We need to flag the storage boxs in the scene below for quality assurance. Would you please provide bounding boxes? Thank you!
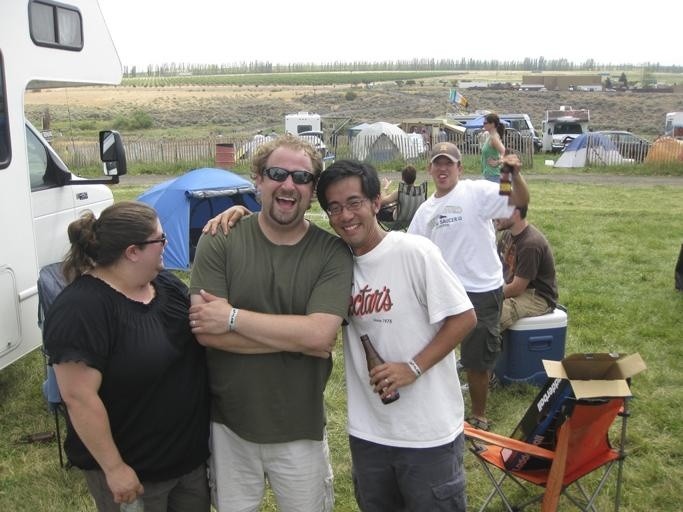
[494,308,568,386]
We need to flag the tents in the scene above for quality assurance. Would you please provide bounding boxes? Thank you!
[135,167,262,271]
[351,122,418,162]
[553,133,623,168]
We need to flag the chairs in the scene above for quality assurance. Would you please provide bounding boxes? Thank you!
[464,378,631,512]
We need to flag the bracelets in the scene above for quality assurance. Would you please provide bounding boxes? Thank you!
[228,308,239,332]
[407,360,422,377]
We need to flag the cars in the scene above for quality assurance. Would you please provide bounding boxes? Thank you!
[299,133,329,157]
[597,129,652,162]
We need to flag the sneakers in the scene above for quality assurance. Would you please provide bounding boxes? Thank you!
[465,418,490,440]
[461,370,500,393]
[456,358,467,371]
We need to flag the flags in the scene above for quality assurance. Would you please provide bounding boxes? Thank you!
[449,88,468,108]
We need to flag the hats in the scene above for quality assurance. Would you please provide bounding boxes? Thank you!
[430,141,462,163]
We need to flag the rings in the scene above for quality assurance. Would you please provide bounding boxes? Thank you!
[192,321,195,326]
[385,379,390,384]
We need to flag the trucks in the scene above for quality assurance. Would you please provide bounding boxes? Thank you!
[665,111,683,141]
[451,108,592,157]
[0,0,133,378]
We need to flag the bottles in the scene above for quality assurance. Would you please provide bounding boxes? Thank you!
[358,334,402,407]
[498,149,512,198]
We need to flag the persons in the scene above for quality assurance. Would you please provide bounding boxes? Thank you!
[40,201,211,512]
[188,133,354,512]
[413,127,447,145]
[203,159,478,512]
[457,204,558,391]
[376,166,416,221]
[480,113,506,183]
[408,141,529,431]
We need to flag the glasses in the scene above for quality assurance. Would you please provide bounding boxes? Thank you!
[125,232,167,249]
[262,166,321,185]
[326,197,370,216]
[484,121,489,125]
